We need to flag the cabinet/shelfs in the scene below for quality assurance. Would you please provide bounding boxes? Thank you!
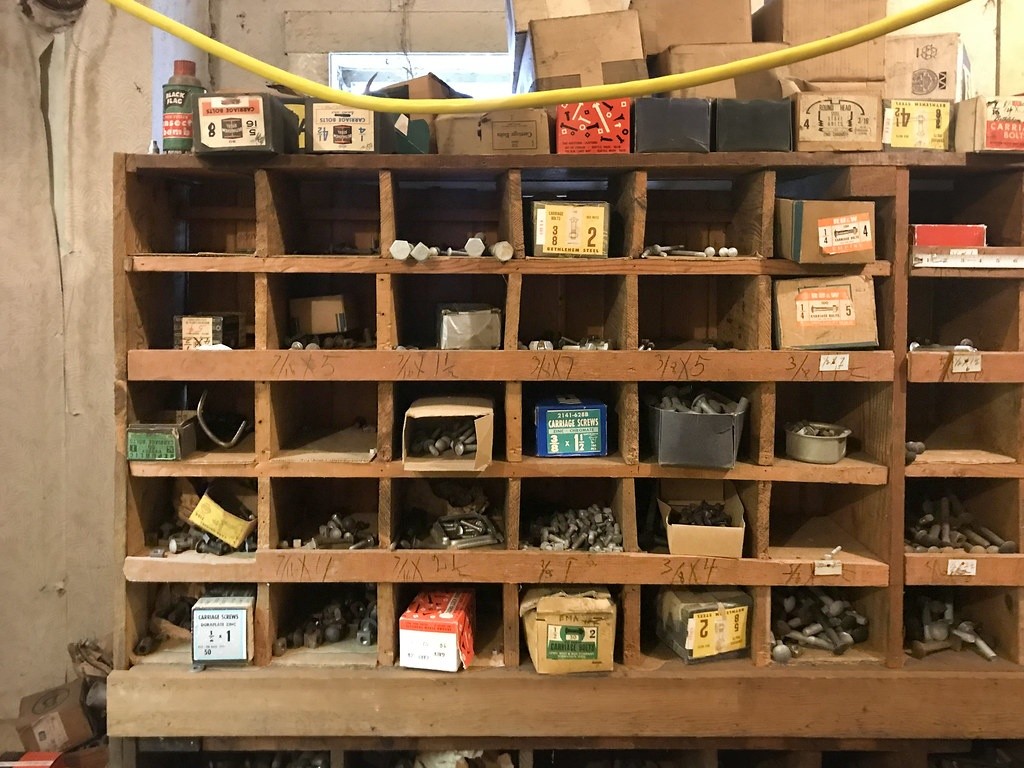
[107,145,1024,768]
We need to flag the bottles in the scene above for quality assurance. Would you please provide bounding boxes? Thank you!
[162,60,203,151]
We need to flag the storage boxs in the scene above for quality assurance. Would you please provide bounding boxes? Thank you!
[192,591,253,665]
[126,408,204,461]
[531,200,611,259]
[656,479,746,557]
[289,293,357,336]
[777,195,880,268]
[173,311,245,350]
[535,393,608,457]
[649,394,749,468]
[766,275,884,353]
[910,223,989,250]
[160,477,259,549]
[192,0,1024,154]
[656,587,754,664]
[401,392,493,471]
[519,587,617,673]
[399,588,475,672]
[434,302,502,350]
